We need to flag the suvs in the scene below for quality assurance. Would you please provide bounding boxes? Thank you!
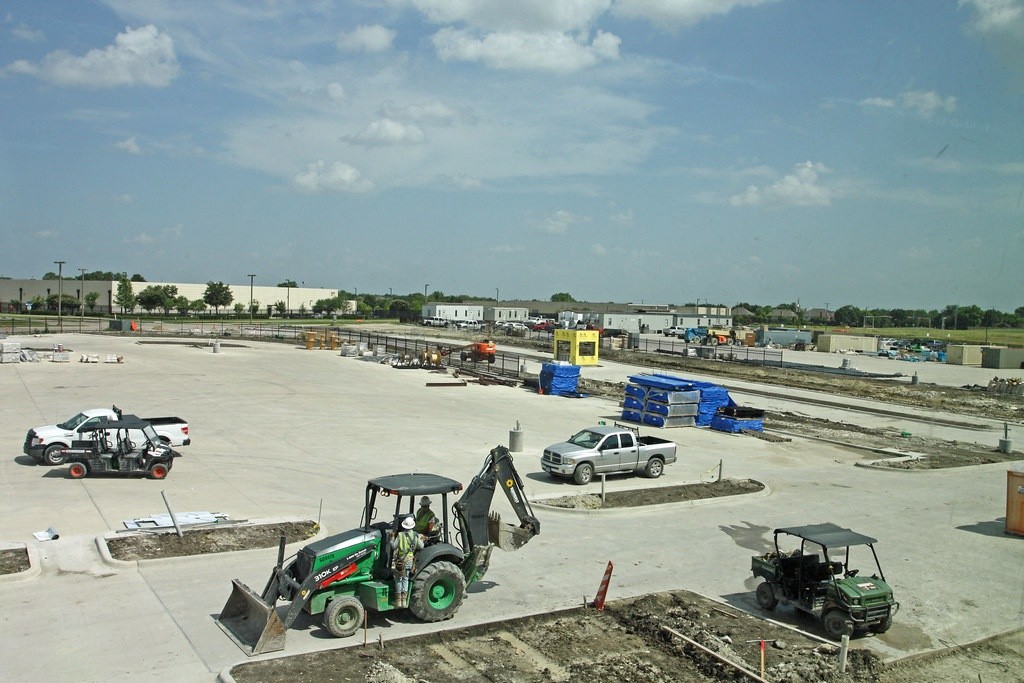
[603,328,632,337]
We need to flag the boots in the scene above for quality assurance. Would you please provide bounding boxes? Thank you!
[401,592,408,608]
[392,593,402,608]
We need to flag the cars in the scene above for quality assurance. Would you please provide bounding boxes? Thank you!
[490,320,529,332]
[874,337,967,351]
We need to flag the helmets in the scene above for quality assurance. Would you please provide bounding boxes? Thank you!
[401,516,416,529]
[419,496,432,506]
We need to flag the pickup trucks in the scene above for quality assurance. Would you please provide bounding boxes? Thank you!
[578,324,604,338]
[421,316,450,327]
[22,405,191,464]
[663,326,686,337]
[456,320,487,331]
[532,321,554,331]
[541,418,678,486]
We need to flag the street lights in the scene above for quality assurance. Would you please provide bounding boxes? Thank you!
[247,274,257,323]
[54,261,66,326]
[285,279,290,319]
[77,268,87,321]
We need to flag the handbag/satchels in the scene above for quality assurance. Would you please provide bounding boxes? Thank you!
[394,559,406,576]
[410,557,418,575]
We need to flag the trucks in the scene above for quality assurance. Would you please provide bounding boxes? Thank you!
[752,523,900,641]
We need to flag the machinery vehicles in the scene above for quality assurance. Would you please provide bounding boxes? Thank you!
[436,336,498,365]
[212,443,541,657]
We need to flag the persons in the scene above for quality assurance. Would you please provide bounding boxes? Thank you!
[413,496,435,533]
[389,517,424,607]
